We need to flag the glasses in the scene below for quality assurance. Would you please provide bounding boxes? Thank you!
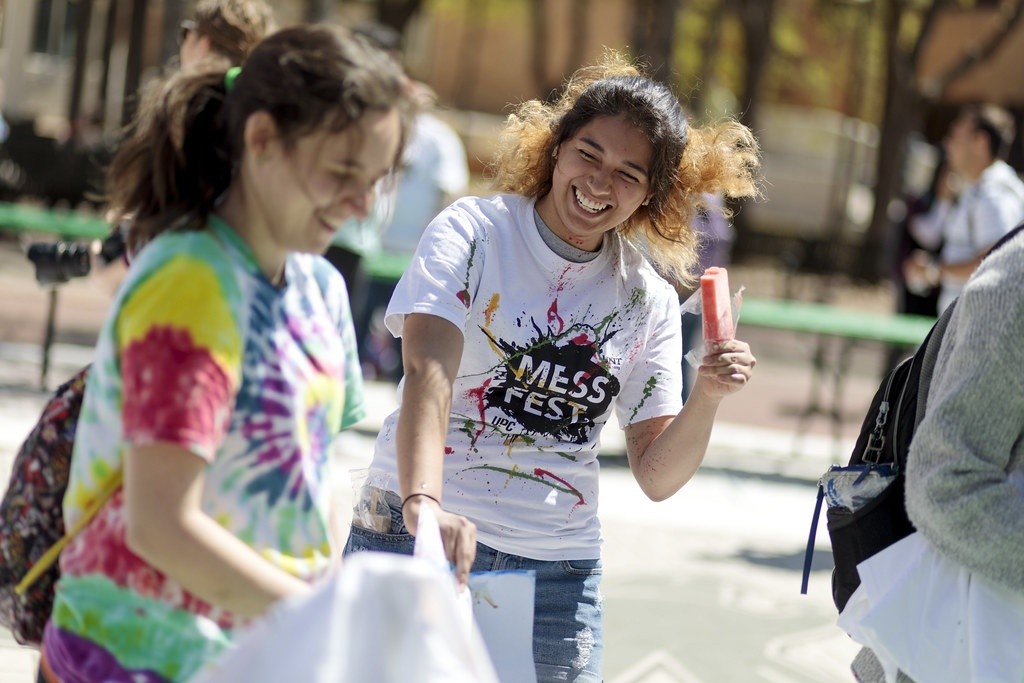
[175,20,197,47]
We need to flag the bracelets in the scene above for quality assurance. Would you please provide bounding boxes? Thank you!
[397,495,439,518]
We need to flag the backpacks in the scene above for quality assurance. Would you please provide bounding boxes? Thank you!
[0,365,122,643]
[820,220,1024,616]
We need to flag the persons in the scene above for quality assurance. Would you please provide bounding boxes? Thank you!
[332,81,479,387]
[910,105,1023,316]
[847,218,1023,683]
[175,1,269,75]
[657,175,724,408]
[36,20,416,683]
[345,62,757,683]
